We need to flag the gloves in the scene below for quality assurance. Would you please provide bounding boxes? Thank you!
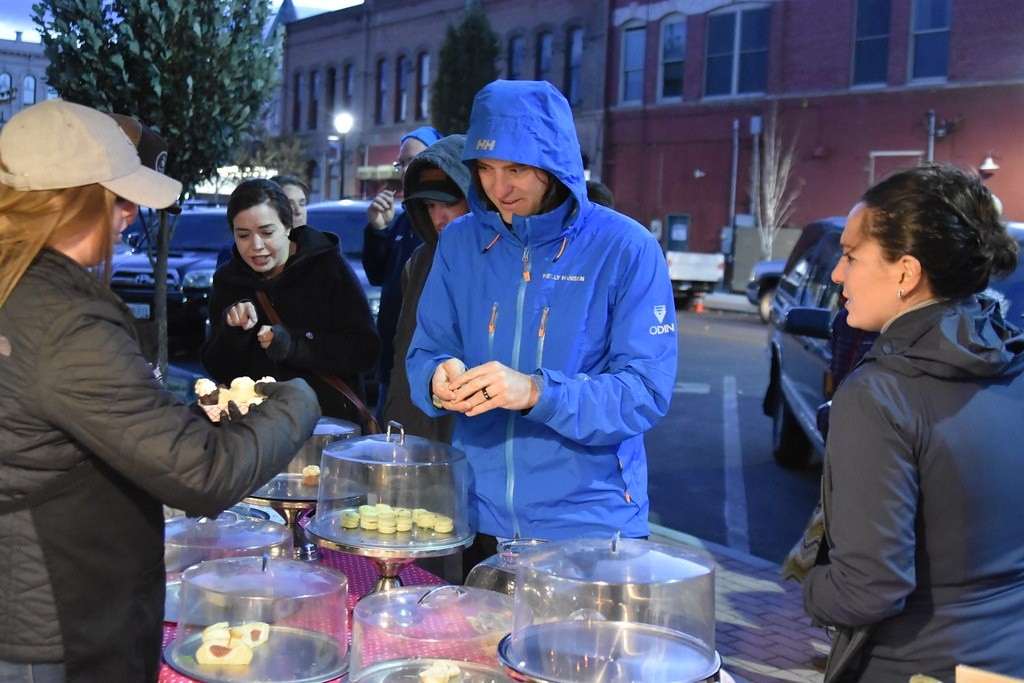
[219,400,257,425]
[255,378,323,415]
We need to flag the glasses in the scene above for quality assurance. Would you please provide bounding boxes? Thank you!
[392,160,411,173]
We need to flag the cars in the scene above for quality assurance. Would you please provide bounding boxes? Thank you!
[303,196,403,326]
[83,199,237,306]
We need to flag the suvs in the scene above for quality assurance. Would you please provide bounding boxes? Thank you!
[761,214,1024,466]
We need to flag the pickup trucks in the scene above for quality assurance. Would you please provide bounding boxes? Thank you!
[662,248,726,312]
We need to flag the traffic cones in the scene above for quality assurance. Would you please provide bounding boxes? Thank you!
[693,298,705,314]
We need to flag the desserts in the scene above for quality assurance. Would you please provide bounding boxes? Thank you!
[420,660,460,683]
[194,376,278,405]
[299,464,454,534]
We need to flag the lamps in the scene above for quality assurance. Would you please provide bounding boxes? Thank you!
[977,148,1000,170]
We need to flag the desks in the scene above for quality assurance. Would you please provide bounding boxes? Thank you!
[157,507,530,683]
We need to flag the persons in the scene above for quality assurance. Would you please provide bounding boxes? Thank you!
[103,114,182,224]
[362,126,446,422]
[201,179,381,437]
[404,79,678,586]
[382,134,472,446]
[801,163,1024,683]
[215,174,309,264]
[0,97,322,683]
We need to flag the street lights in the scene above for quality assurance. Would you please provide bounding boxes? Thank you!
[333,110,354,200]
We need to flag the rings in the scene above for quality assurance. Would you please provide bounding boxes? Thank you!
[482,388,491,400]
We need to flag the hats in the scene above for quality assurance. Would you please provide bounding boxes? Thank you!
[0,98,183,210]
[116,124,183,214]
[402,164,464,203]
[585,181,614,206]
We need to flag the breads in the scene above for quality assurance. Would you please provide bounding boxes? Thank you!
[194,622,270,665]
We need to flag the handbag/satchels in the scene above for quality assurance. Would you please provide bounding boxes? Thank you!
[363,415,383,435]
